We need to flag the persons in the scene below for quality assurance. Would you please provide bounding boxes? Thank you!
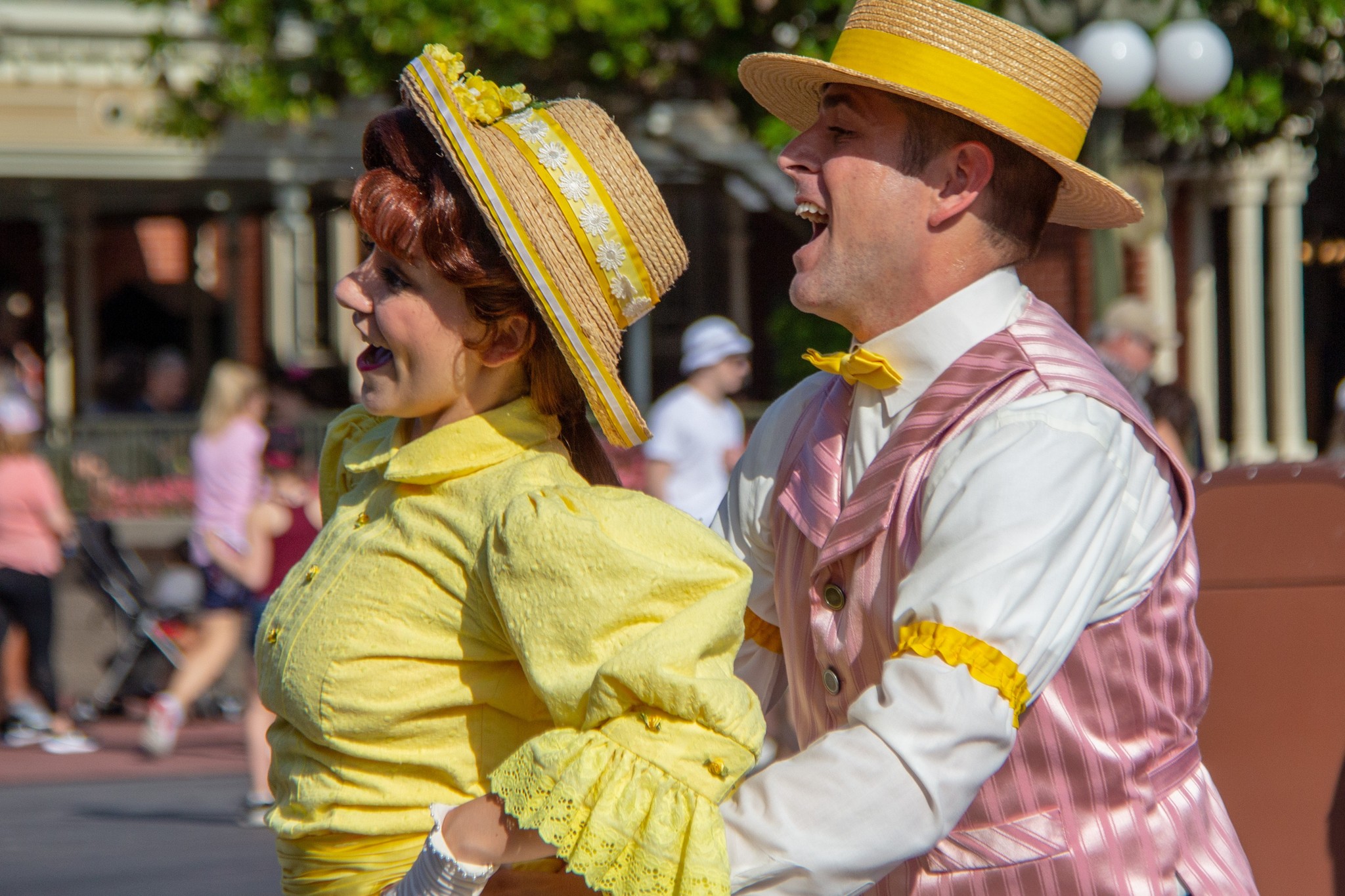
[641,316,755,530]
[144,360,270,756]
[253,44,768,896]
[1090,291,1209,478]
[197,433,323,826]
[481,1,1256,895]
[0,392,97,754]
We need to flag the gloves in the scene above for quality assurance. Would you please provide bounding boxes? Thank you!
[381,804,499,895]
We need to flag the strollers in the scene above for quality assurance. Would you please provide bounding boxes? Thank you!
[62,510,246,729]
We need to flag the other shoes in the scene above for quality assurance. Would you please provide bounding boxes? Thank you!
[140,692,185,763]
[43,727,100,754]
[7,720,58,748]
[241,792,279,830]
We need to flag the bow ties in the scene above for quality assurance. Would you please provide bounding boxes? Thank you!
[801,342,903,391]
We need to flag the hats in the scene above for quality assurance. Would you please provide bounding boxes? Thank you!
[1103,293,1164,352]
[400,42,691,451]
[736,0,1146,231]
[680,316,754,373]
